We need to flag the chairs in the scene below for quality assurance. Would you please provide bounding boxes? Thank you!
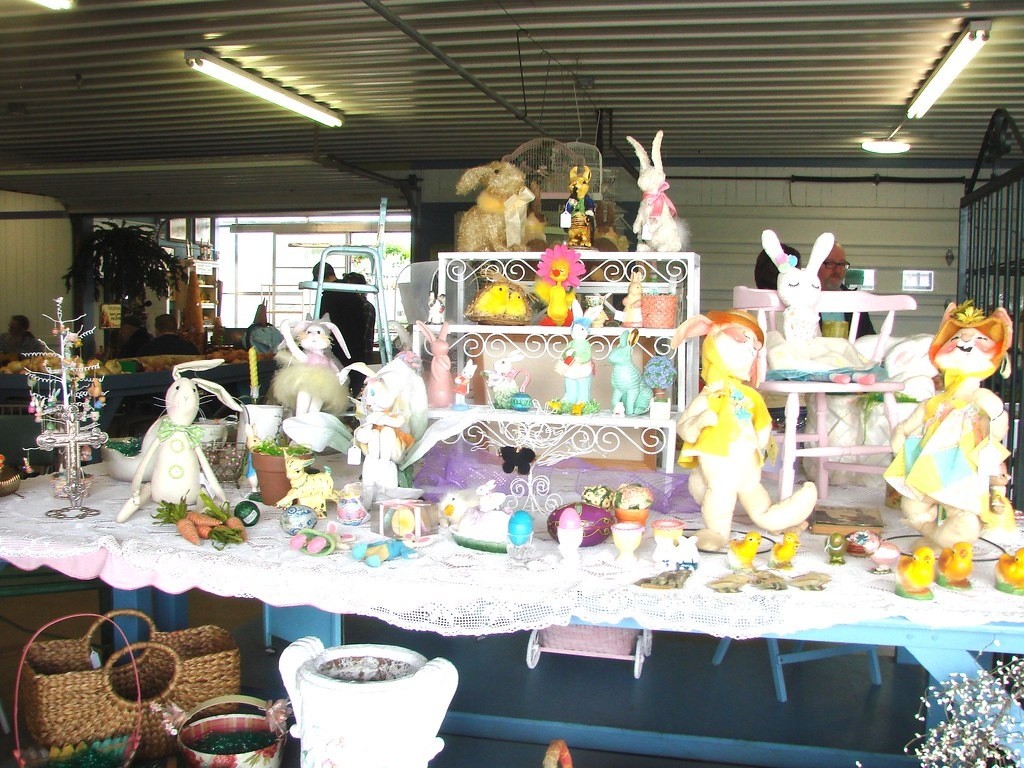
[732,285,916,504]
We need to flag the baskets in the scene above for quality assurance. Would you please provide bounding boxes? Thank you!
[641,296,677,329]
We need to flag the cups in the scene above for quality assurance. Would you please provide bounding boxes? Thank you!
[614,509,649,527]
[558,525,584,562]
[653,520,685,547]
[584,295,604,308]
[506,532,533,562]
[822,321,849,340]
[611,523,644,564]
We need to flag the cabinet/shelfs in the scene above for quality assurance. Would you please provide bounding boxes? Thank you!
[175,258,220,350]
[413,251,701,510]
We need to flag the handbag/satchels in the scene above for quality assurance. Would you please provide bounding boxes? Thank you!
[20,610,240,768]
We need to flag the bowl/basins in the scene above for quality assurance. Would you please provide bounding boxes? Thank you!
[101,447,157,481]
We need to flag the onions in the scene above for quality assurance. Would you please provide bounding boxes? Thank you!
[0,349,274,376]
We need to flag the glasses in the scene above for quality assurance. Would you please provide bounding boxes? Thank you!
[823,259,850,271]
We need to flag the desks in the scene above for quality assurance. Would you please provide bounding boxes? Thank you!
[0,460,1024,768]
[0,358,284,464]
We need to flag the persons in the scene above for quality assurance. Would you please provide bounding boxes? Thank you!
[0,314,199,367]
[312,262,376,434]
[754,240,877,433]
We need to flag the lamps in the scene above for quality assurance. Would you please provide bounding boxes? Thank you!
[186,52,348,135]
[906,20,995,121]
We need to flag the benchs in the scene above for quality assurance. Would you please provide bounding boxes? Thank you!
[126,355,205,369]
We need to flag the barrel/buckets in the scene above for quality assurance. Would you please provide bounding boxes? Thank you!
[177,695,289,768]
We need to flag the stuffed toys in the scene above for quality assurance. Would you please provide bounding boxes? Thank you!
[456,161,551,252]
[438,488,481,528]
[351,533,433,568]
[289,521,356,558]
[626,130,691,252]
[476,479,506,512]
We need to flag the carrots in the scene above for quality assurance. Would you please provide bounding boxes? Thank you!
[175,511,248,547]
